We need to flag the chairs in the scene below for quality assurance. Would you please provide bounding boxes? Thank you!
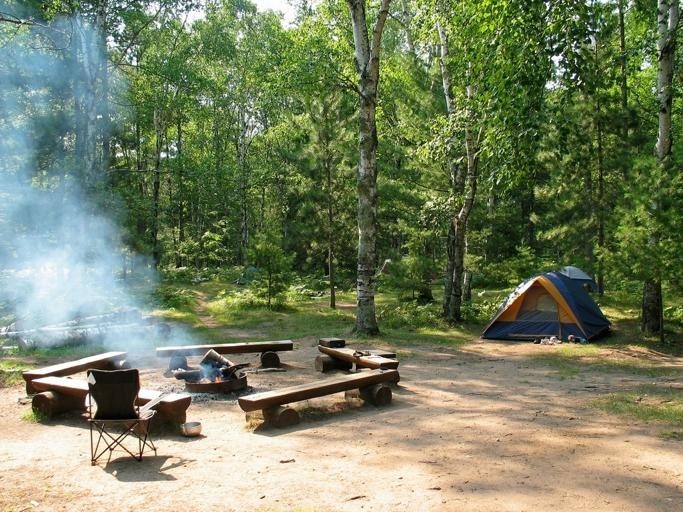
[82,368,161,466]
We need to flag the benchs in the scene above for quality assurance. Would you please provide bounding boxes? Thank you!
[29,376,191,426]
[19,350,130,396]
[239,368,402,427]
[321,337,400,370]
[154,340,293,375]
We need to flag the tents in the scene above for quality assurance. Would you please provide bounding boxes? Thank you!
[480,266,613,343]
